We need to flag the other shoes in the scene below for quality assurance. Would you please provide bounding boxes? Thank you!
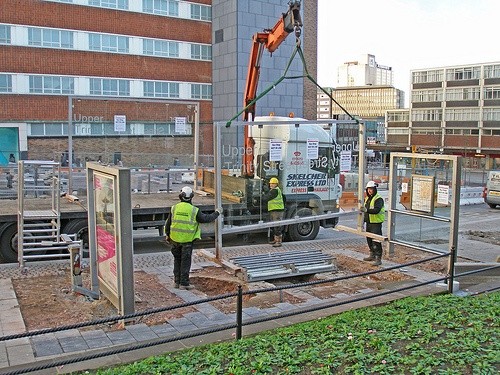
[269,235,282,247]
[363,251,376,261]
[173,282,195,290]
[371,256,382,266]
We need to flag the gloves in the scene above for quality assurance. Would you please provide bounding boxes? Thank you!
[360,206,368,213]
[215,206,223,215]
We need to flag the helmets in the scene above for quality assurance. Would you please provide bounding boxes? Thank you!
[268,177,279,184]
[367,181,377,188]
[181,186,192,198]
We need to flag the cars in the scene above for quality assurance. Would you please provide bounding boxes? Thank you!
[482,171,500,208]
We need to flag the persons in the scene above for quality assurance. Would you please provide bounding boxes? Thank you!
[82,156,88,167]
[258,177,285,247]
[360,181,385,266]
[61,153,66,167]
[174,156,181,166]
[164,186,223,290]
[75,158,80,168]
[97,156,102,162]
[116,158,123,167]
[6,172,13,188]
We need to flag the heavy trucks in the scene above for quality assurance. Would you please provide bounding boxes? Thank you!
[0,0,343,265]
